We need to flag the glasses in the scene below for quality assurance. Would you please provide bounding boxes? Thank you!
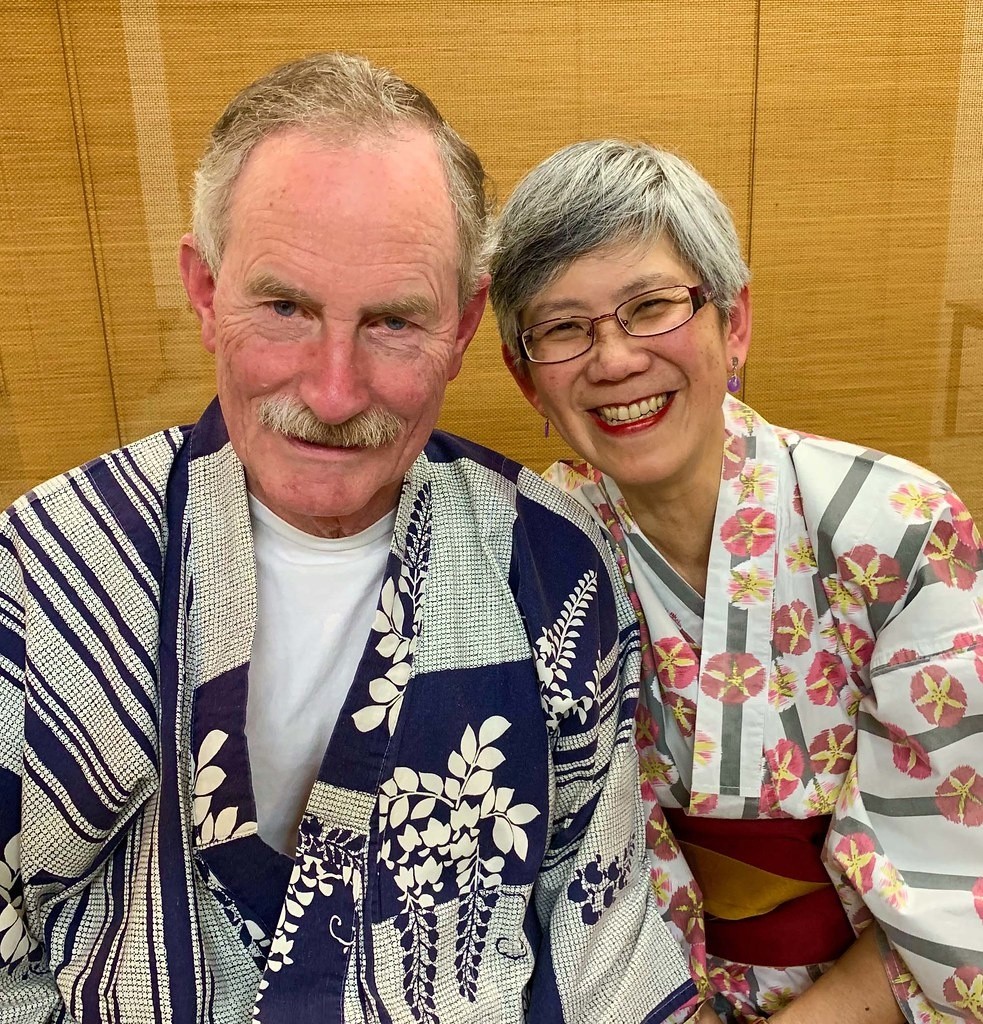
[518,282,713,364]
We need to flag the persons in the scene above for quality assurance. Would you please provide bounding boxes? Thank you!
[0,54,695,1024]
[494,141,983,1024]
